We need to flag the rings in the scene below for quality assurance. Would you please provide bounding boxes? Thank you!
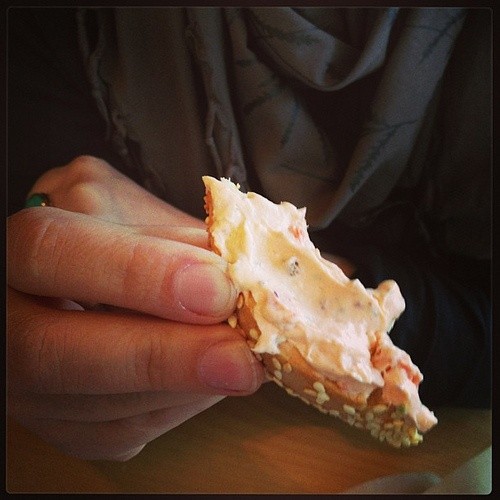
[22,190,53,212]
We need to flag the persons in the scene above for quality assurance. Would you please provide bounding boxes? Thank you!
[0,0,494,472]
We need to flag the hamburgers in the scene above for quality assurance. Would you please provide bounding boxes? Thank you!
[199,172,438,450]
